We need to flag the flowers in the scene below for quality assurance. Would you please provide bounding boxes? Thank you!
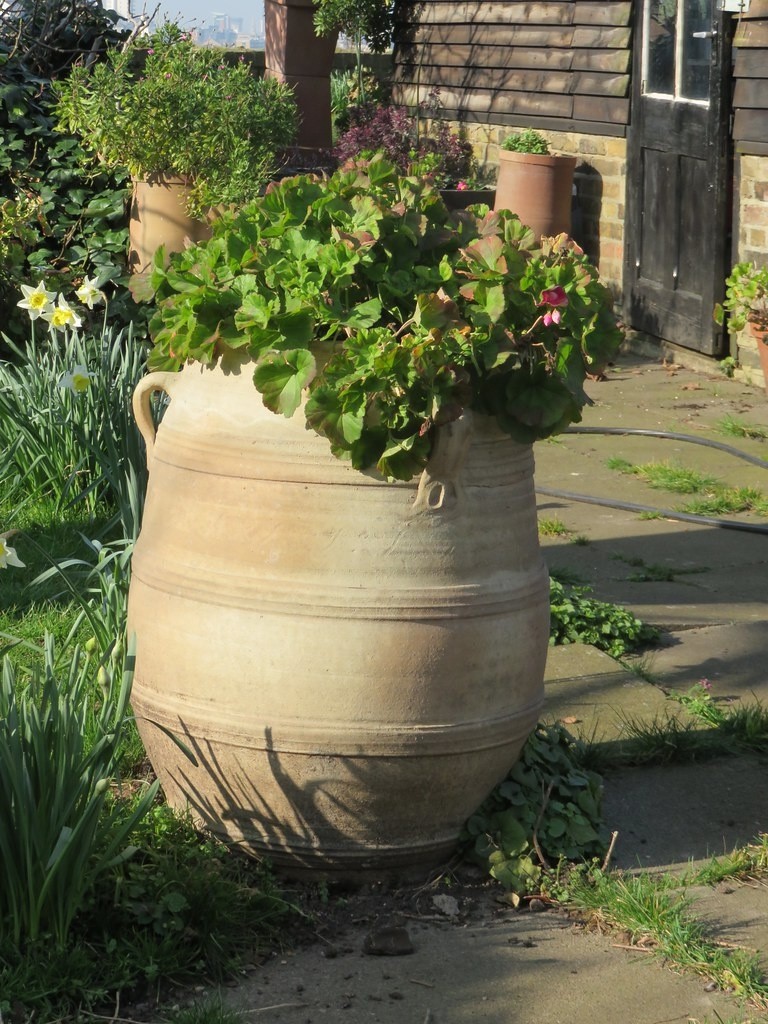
[49,15,304,222]
[127,149,623,486]
[456,181,470,191]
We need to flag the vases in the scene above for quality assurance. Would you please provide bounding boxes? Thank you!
[126,168,257,306]
[133,331,550,882]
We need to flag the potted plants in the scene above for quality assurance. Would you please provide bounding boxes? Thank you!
[493,126,577,245]
[328,85,496,214]
[714,263,768,400]
